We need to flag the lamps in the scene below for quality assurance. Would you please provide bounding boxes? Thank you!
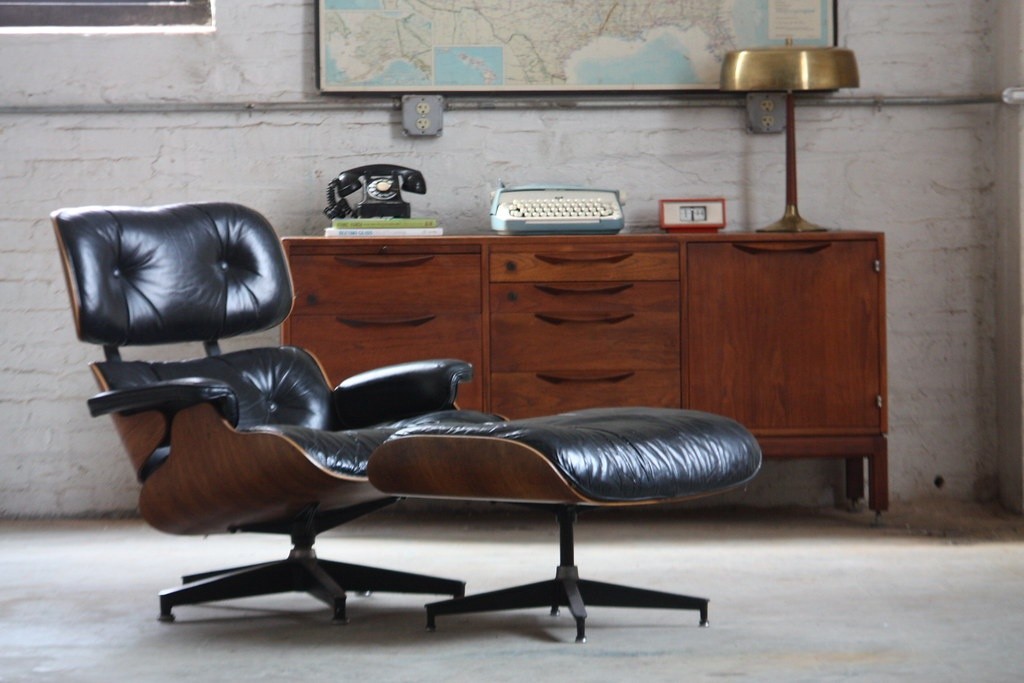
[717,38,862,233]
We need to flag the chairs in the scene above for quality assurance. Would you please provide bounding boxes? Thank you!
[50,199,511,626]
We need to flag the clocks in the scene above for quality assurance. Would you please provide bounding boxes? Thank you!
[657,197,728,233]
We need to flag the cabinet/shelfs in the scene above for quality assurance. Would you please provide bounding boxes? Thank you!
[280,230,890,528]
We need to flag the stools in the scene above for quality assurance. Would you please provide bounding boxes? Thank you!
[366,405,765,645]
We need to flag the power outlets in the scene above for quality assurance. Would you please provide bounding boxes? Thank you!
[400,94,444,137]
[743,91,790,134]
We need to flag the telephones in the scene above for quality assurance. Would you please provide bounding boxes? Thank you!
[337,164,427,218]
